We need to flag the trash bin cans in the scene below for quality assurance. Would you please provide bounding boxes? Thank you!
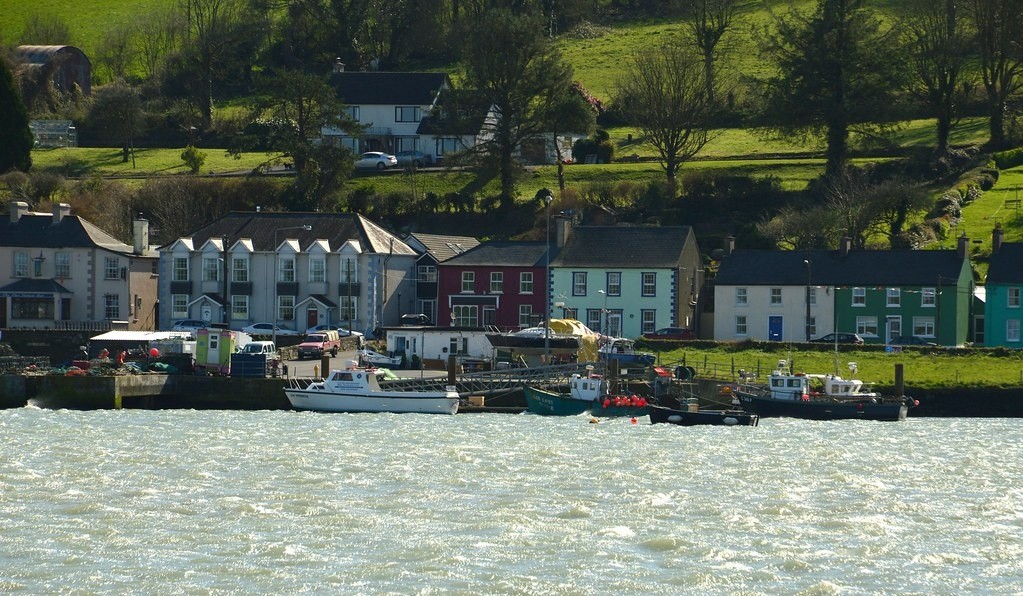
[462,360,491,373]
[886,345,902,352]
[687,403,700,412]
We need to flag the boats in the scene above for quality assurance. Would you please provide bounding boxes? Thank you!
[282,360,461,416]
[361,348,404,368]
[484,199,920,425]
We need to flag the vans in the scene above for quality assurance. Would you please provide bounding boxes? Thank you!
[242,340,276,359]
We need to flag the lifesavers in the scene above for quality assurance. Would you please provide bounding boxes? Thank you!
[591,373,603,379]
[365,367,377,372]
[794,373,807,376]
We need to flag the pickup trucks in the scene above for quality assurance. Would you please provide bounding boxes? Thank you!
[297,330,341,360]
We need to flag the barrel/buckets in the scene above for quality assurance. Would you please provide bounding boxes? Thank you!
[687,404,699,412]
[468,396,485,406]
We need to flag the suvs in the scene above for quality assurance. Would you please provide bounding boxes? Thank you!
[809,333,865,346]
[642,326,698,340]
[170,319,212,341]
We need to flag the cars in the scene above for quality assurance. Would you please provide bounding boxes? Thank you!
[889,334,938,347]
[305,324,364,337]
[395,150,432,168]
[353,151,398,170]
[241,322,299,336]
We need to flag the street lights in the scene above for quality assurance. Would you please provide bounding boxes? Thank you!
[273,225,312,333]
